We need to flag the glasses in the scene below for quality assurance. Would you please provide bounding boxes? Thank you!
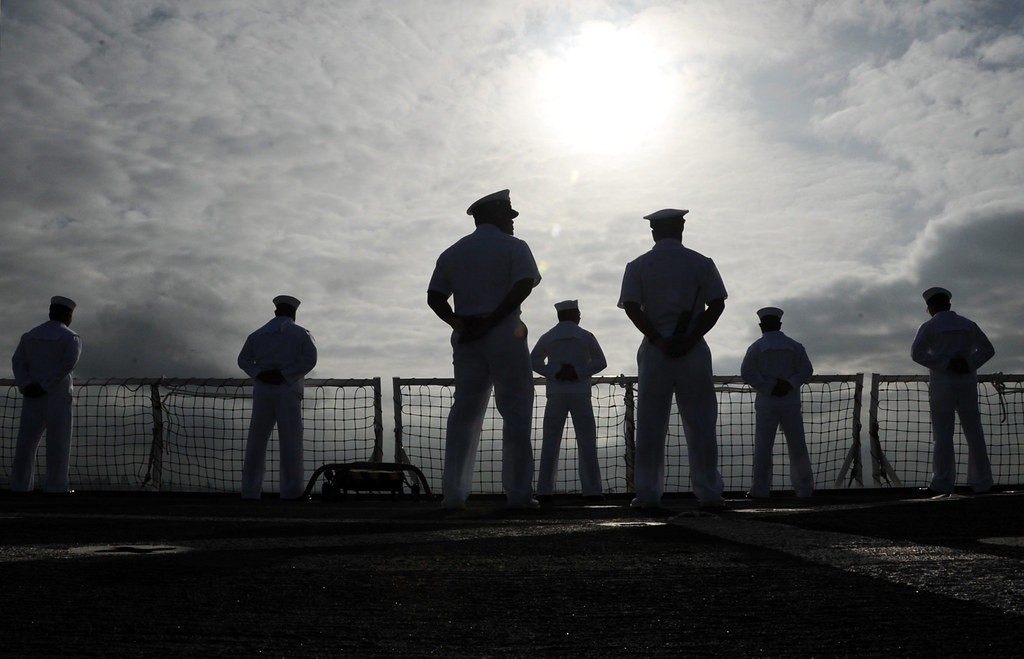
[926,306,930,313]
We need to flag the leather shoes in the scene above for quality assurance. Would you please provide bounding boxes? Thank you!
[915,487,954,497]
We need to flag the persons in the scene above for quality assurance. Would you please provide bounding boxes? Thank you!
[427,186,543,515]
[911,286,998,496]
[620,206,730,516]
[533,299,609,500]
[11,295,82,501]
[742,305,816,507]
[237,295,318,505]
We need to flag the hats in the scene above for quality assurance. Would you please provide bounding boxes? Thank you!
[642,209,689,227]
[50,295,77,312]
[922,287,952,302]
[554,299,579,311]
[756,307,784,320]
[273,294,302,311]
[466,189,518,220]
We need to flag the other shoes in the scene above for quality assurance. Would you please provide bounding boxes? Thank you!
[241,493,261,502]
[440,499,465,511]
[536,494,554,502]
[11,489,38,496]
[508,498,541,511]
[280,494,312,501]
[583,494,606,502]
[630,496,657,507]
[745,491,772,500]
[699,500,728,509]
